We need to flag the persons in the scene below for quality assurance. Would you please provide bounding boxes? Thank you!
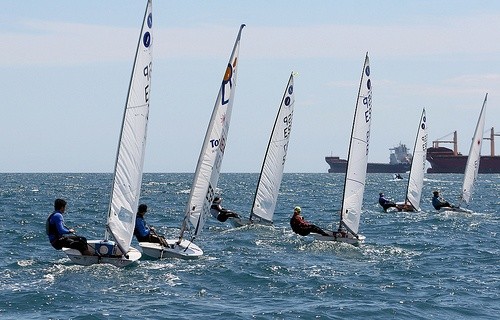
[290,206,329,236]
[46,198,92,256]
[431,191,452,210]
[377,192,398,210]
[209,196,242,223]
[133,203,162,243]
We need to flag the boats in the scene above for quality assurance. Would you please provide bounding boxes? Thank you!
[325,157,407,173]
[425,127,500,173]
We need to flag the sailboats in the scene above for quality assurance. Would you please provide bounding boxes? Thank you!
[139,24,246,260]
[230,71,295,227]
[311,51,372,246]
[440,93,488,213]
[391,108,428,212]
[62,0,142,266]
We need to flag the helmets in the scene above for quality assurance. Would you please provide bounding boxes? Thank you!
[294,206,301,213]
